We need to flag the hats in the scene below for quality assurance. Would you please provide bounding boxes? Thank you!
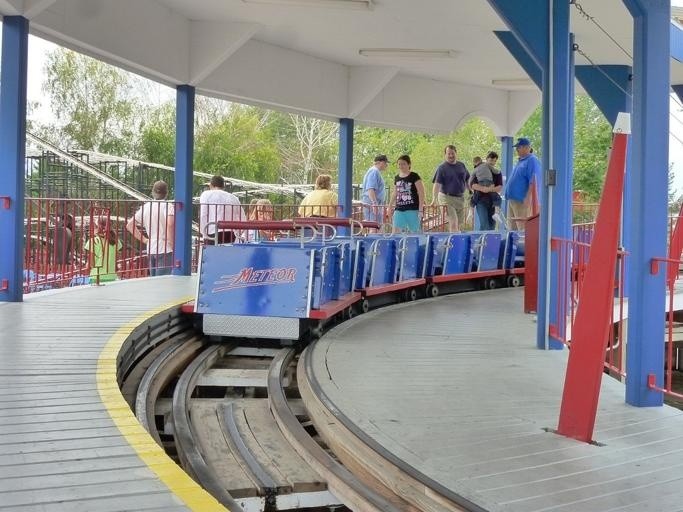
[152,180,168,194]
[471,156,482,165]
[373,154,391,164]
[511,138,530,147]
[211,175,224,187]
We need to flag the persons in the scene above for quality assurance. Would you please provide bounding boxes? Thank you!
[83,216,122,283]
[298,175,372,217]
[240,199,272,244]
[471,151,503,231]
[468,157,503,224]
[200,176,247,244]
[125,180,175,275]
[430,145,474,231]
[504,137,542,232]
[362,155,392,233]
[387,155,425,232]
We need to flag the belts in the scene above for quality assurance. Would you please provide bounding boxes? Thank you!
[439,191,462,197]
